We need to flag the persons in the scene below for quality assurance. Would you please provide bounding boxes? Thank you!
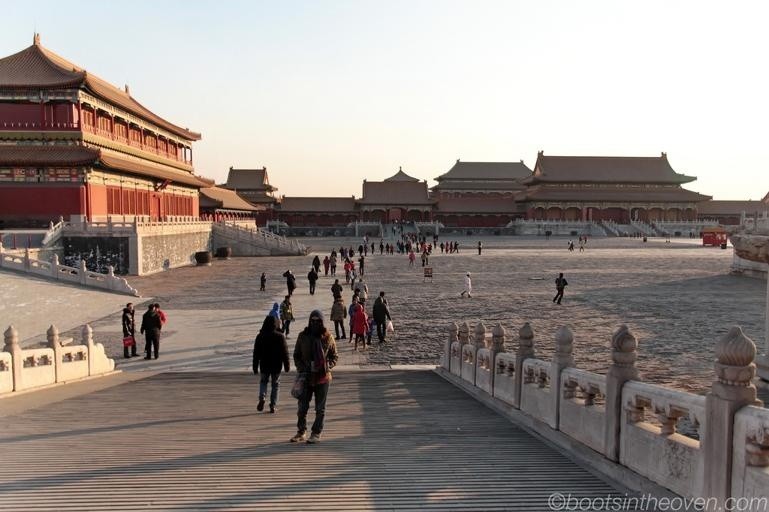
[566,233,587,253]
[260,231,393,353]
[477,241,483,255]
[289,309,338,445]
[252,314,290,413]
[461,270,473,299]
[552,272,569,304]
[391,217,460,267]
[122,302,167,360]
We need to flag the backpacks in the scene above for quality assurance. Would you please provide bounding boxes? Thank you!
[301,335,327,378]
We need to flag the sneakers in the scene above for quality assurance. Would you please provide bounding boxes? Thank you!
[257,398,275,413]
[291,434,321,444]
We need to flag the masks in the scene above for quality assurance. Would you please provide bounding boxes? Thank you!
[309,321,322,328]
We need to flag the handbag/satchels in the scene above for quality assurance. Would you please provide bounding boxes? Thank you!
[123,336,135,347]
[291,375,306,398]
